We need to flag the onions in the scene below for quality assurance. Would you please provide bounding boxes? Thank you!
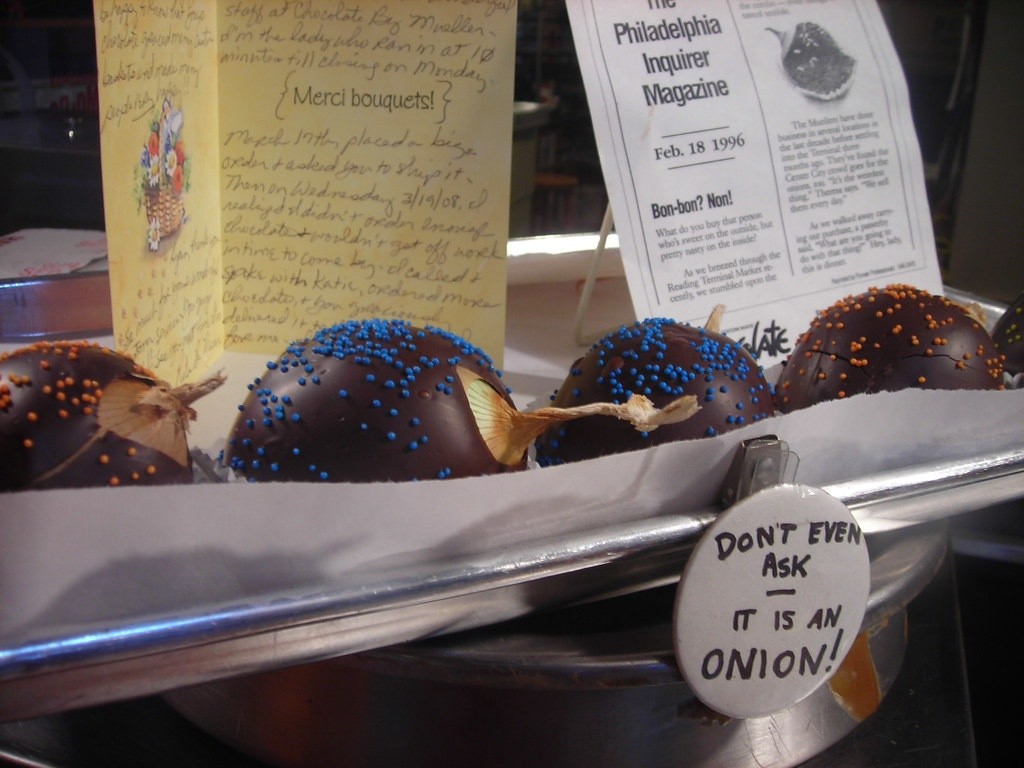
[227,320,702,483]
[534,281,1024,465]
[0,340,224,492]
